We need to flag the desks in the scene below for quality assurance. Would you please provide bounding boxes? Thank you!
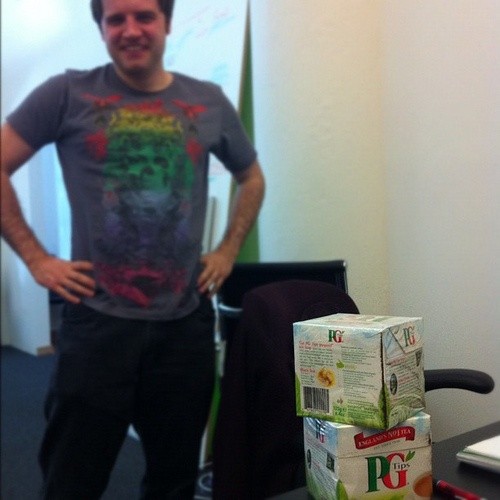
[268,421,500,500]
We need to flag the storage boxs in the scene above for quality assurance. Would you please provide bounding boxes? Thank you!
[302,411,433,500]
[293,312,427,432]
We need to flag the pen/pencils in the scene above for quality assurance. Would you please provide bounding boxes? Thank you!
[432,477,487,500]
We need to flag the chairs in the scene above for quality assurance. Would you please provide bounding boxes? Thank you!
[212,260,494,500]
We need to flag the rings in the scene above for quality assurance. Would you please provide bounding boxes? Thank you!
[208,282,215,293]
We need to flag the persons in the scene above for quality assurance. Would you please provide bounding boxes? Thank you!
[1,0,266,500]
